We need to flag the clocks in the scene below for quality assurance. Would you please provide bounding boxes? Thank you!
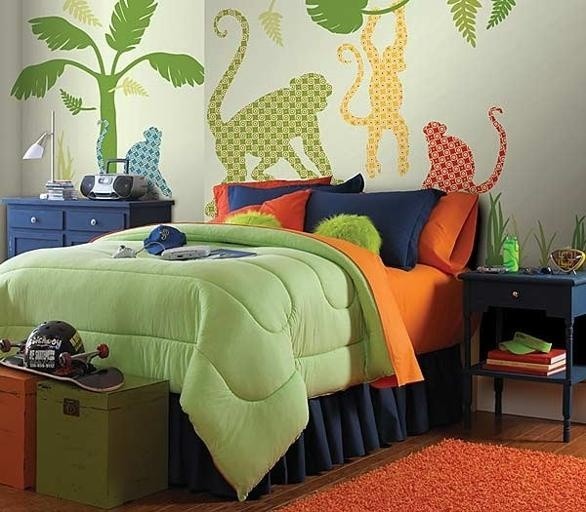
[547,246,584,277]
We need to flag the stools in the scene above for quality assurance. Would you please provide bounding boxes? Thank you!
[0,366,170,510]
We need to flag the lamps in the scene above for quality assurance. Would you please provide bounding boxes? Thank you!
[21,109,55,183]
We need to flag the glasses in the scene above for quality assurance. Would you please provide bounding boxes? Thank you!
[531,265,571,276]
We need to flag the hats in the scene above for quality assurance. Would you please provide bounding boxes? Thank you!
[498,332,552,355]
[144,225,187,256]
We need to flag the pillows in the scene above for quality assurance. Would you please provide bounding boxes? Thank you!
[208,171,478,277]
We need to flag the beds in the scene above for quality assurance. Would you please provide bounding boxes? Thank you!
[0,202,485,501]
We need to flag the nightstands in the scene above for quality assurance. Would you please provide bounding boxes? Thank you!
[451,267,585,443]
[0,193,174,261]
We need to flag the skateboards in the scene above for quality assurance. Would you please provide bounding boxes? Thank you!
[0,337,126,393]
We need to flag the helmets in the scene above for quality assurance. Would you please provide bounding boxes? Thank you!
[23,320,85,377]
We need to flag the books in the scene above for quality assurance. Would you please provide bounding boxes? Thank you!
[480,347,567,380]
[190,247,257,261]
[46,180,75,201]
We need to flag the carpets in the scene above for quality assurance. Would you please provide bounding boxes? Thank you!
[264,439,586,512]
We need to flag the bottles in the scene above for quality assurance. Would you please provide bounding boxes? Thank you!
[502,235,520,271]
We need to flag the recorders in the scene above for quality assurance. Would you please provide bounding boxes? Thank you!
[79,157,147,201]
